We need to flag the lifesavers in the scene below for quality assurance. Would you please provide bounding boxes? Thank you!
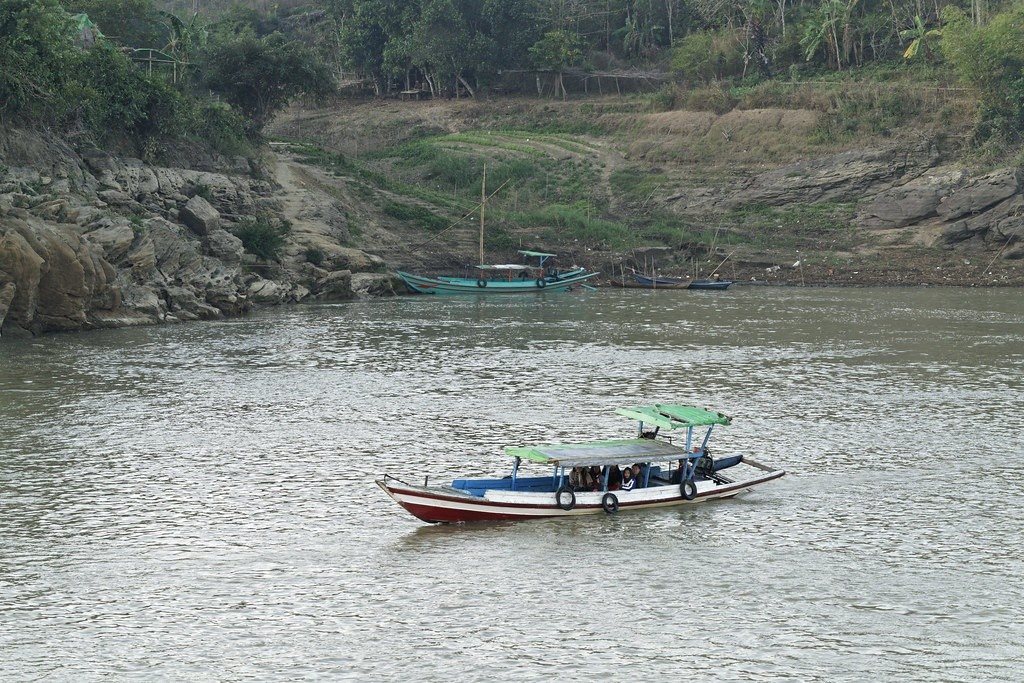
[602,493,619,513]
[518,272,529,278]
[680,479,697,500]
[536,278,546,288]
[477,279,487,287]
[557,487,576,511]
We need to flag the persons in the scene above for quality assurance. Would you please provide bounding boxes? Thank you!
[569,464,644,492]
[678,459,695,480]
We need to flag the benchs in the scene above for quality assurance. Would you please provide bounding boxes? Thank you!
[647,479,670,487]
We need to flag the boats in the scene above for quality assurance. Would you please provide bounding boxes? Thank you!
[633,273,732,289]
[395,250,600,294]
[375,403,785,523]
